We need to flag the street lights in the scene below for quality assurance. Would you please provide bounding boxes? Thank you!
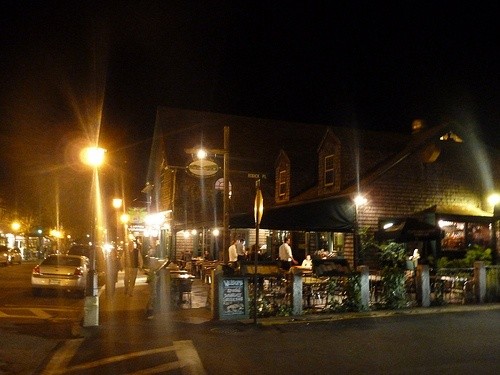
[111,192,122,272]
[211,228,221,262]
[82,143,105,327]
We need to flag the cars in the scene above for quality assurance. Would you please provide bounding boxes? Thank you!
[0,245,23,267]
[29,253,89,298]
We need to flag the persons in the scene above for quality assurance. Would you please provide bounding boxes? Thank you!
[121,242,143,296]
[302,255,312,266]
[237,239,246,260]
[229,240,238,268]
[205,251,214,261]
[183,250,192,260]
[148,245,154,257]
[279,237,298,280]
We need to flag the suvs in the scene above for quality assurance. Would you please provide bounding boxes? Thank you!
[69,243,109,284]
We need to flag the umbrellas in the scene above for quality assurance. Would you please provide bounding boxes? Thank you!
[375,218,445,243]
